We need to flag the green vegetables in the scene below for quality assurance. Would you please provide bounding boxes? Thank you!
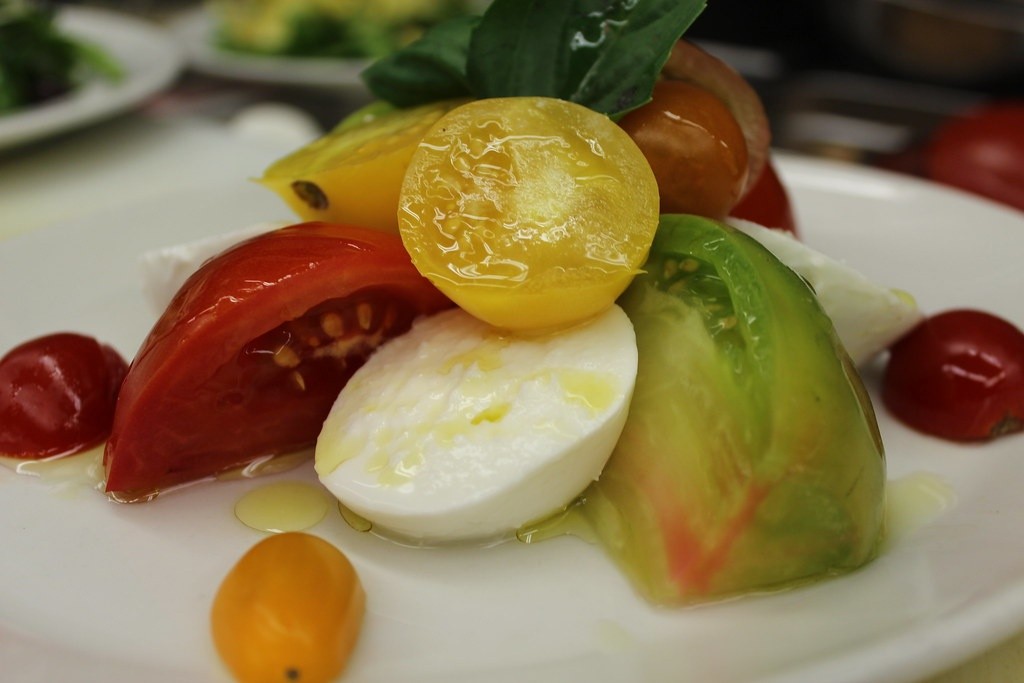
[357,0,709,118]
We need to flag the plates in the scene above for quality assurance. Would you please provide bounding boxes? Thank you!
[169,11,379,95]
[1,151,1024,683]
[0,8,184,147]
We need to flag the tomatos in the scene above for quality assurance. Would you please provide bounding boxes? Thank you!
[0,39,1024,683]
